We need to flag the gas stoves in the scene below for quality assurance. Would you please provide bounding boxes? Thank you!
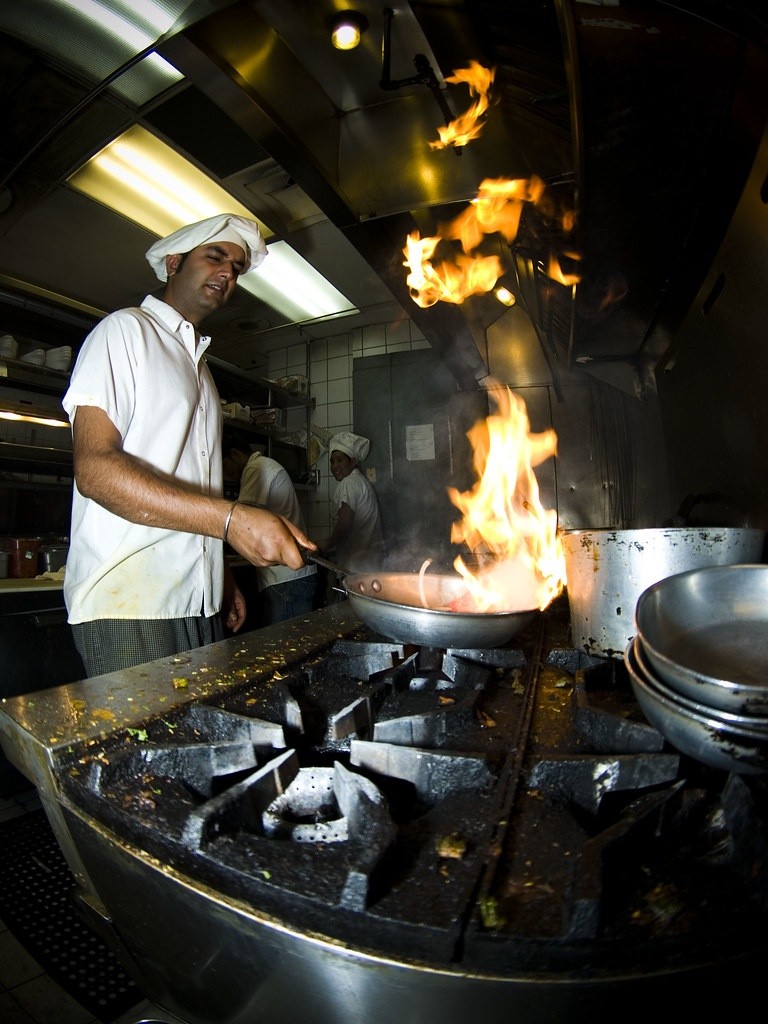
[0,584,768,1024]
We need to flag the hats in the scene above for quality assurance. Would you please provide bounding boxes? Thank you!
[146,213,269,282]
[329,430,371,464]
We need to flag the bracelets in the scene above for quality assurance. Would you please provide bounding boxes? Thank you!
[223,499,238,542]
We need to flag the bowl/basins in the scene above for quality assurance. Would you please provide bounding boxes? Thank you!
[43,345,72,370]
[0,334,19,359]
[19,348,46,366]
[557,525,768,663]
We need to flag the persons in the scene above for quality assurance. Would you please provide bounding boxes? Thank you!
[314,432,383,603]
[222,437,318,625]
[61,213,318,683]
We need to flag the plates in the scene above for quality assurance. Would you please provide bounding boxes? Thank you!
[624,563,768,775]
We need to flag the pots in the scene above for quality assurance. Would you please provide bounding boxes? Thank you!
[293,532,568,652]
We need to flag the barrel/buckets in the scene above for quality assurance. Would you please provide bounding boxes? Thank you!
[37,542,70,574]
[4,535,40,579]
